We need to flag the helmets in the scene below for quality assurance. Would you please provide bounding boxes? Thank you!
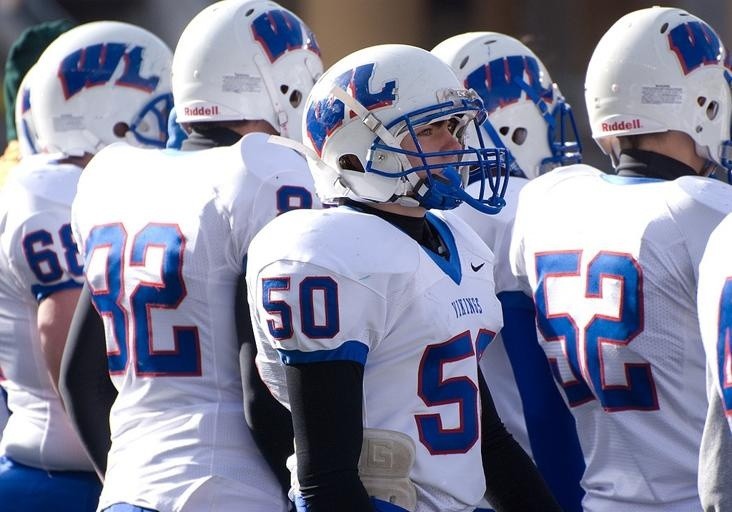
[431,28,582,175]
[28,21,171,163]
[14,71,41,160]
[172,0,322,141]
[583,4,732,170]
[301,44,511,214]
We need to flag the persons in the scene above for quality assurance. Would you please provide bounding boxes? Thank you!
[0,16,77,187]
[691,212,731,512]
[15,62,48,158]
[0,21,172,512]
[430,32,589,510]
[55,0,328,512]
[245,45,560,511]
[510,6,732,512]
